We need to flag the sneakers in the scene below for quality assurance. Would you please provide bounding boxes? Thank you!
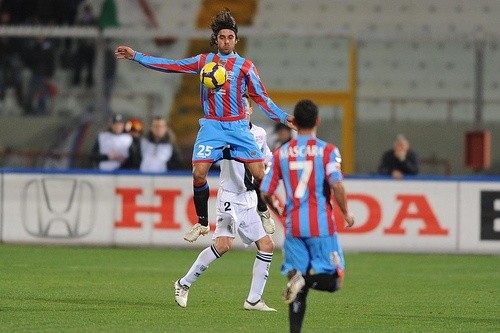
[183,223,210,242]
[173,279,191,308]
[256,204,276,234]
[243,299,277,312]
[284,269,305,303]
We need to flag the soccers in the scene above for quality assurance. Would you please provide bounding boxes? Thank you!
[200,62,226,88]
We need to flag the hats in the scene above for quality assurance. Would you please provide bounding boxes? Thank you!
[112,112,125,124]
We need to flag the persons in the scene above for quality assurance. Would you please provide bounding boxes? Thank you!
[114,8,298,242]
[0,0,418,180]
[173,95,277,312]
[260,100,354,333]
